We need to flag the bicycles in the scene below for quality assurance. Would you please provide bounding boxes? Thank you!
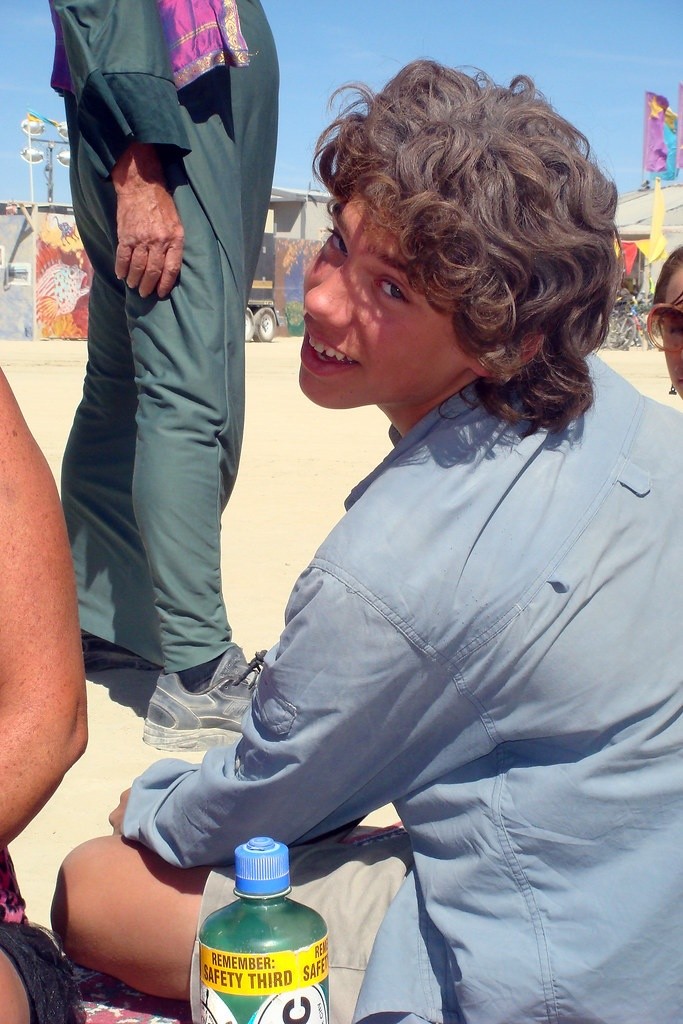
[598,286,657,351]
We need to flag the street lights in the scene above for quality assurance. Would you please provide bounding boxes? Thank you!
[20,118,70,203]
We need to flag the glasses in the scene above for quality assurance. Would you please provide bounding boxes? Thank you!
[646,302,683,352]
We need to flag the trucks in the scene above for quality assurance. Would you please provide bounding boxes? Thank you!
[245,232,280,343]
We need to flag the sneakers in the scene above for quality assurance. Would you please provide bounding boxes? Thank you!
[76,629,172,675]
[142,644,273,753]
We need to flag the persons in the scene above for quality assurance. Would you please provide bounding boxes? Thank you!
[646,247,683,401]
[50,58,683,1024]
[0,369,89,1024]
[48,0,279,747]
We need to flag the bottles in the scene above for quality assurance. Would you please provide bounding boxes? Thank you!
[197,835,330,1023]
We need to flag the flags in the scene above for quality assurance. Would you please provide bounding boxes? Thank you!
[642,81,683,181]
